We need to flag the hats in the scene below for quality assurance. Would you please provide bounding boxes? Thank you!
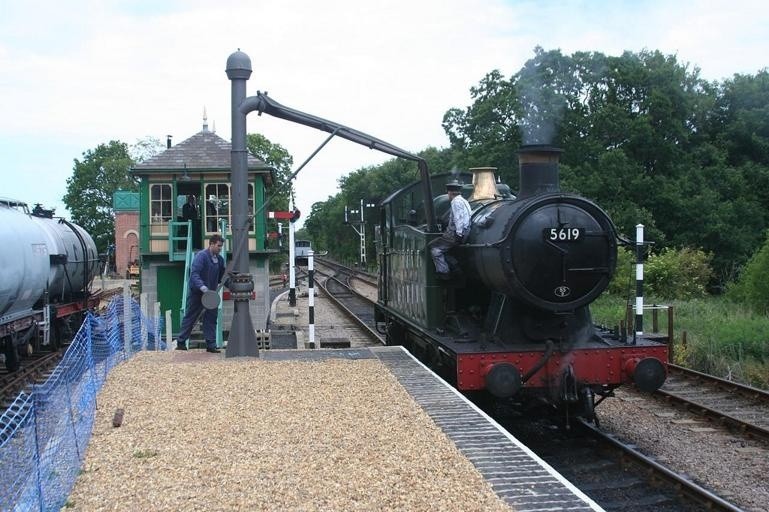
[445,183,462,192]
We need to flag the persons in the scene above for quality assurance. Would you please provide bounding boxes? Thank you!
[199,193,217,232]
[430,183,472,279]
[177,234,228,353]
[182,194,199,248]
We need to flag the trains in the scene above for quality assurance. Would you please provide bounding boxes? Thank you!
[376,144,670,429]
[0,198,100,377]
[295,239,311,260]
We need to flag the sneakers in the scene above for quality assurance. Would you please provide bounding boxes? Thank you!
[432,272,450,280]
[178,341,187,350]
[207,347,220,353]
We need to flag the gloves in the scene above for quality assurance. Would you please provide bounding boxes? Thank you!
[456,235,463,242]
[200,286,208,293]
